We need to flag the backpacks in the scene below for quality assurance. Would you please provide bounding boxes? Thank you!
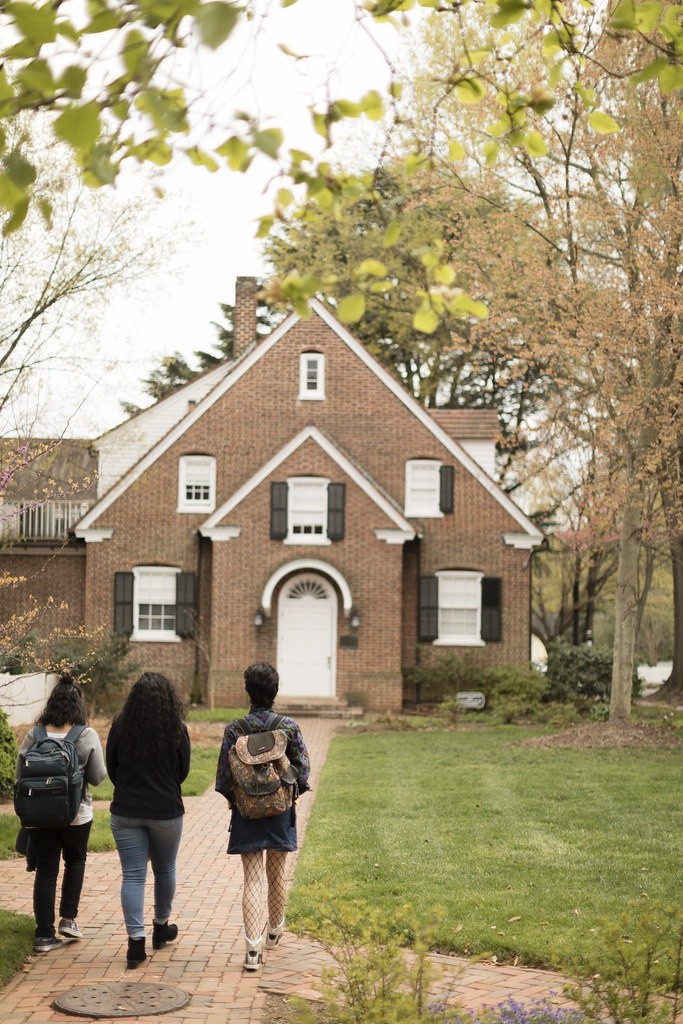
[13,725,85,828]
[228,715,299,819]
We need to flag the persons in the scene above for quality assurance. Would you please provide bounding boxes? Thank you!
[213,662,312,971]
[13,673,107,954]
[105,671,191,970]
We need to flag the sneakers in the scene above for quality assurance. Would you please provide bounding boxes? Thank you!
[34,936,64,951]
[58,919,82,939]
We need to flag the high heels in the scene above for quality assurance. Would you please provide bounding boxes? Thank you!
[126,936,147,969]
[152,920,177,949]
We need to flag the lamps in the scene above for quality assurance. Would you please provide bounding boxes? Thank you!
[254,605,265,630]
[348,604,360,631]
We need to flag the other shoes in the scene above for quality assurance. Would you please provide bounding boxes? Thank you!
[244,936,261,970]
[261,916,284,951]
